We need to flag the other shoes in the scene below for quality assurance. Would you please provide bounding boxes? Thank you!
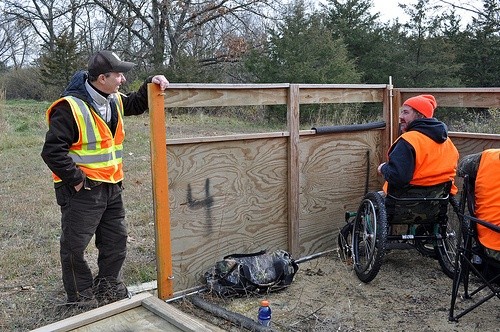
[70,287,98,308]
[95,276,128,299]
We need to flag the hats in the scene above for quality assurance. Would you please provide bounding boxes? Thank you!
[403,94,437,119]
[88,51,134,75]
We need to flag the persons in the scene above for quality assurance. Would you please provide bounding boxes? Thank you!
[377,95,460,235]
[41,50,169,311]
[457,148,500,265]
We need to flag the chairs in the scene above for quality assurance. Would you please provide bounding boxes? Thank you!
[448,170,500,323]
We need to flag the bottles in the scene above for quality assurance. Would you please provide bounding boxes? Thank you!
[257,300,271,328]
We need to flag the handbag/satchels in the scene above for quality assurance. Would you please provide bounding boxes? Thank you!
[203,248,299,298]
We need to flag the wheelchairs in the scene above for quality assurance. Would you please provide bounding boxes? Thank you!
[338,175,470,283]
